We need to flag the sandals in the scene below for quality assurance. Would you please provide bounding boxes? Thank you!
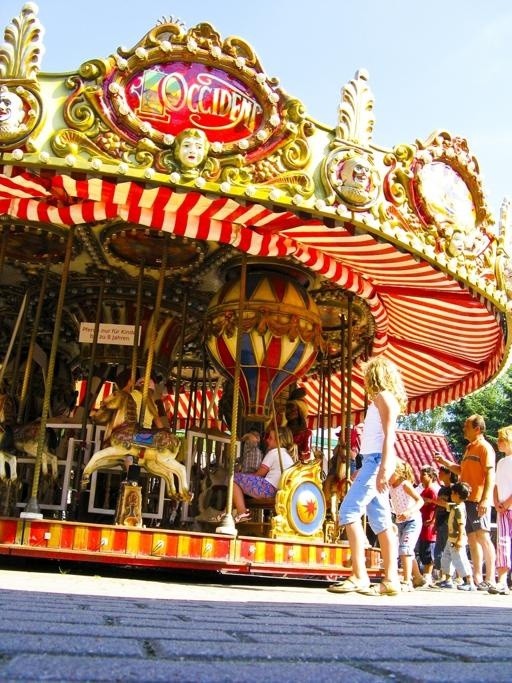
[360,583,398,596]
[327,578,370,592]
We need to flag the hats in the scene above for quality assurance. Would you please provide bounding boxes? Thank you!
[135,377,154,390]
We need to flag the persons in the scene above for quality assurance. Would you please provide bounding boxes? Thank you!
[392,414,511,595]
[267,388,311,460]
[233,428,293,525]
[124,377,164,430]
[328,356,407,598]
[174,127,209,166]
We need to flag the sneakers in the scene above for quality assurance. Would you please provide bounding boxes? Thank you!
[401,578,510,595]
[208,512,226,522]
[234,509,252,523]
